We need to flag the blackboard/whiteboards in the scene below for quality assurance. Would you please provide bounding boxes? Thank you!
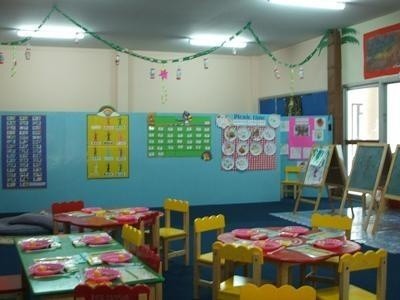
[346,142,388,194]
[383,144,400,201]
[302,143,335,188]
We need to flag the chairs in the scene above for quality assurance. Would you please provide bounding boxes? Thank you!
[280,166,302,199]
